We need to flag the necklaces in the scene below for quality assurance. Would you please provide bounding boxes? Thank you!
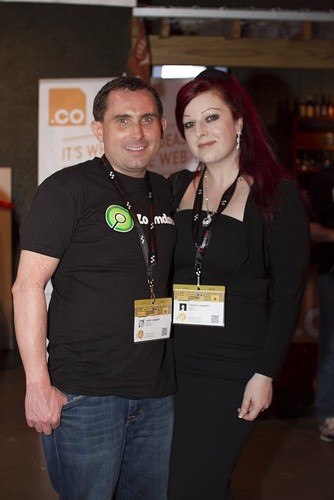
[201,167,231,223]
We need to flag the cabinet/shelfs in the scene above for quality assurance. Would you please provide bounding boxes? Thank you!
[283,113,334,184]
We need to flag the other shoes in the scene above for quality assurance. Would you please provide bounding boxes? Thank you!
[319,417,334,442]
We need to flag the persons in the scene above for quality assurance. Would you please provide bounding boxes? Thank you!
[168,71,334,499]
[10,75,171,499]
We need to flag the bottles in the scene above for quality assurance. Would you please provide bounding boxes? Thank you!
[279,94,334,131]
[294,158,330,169]
